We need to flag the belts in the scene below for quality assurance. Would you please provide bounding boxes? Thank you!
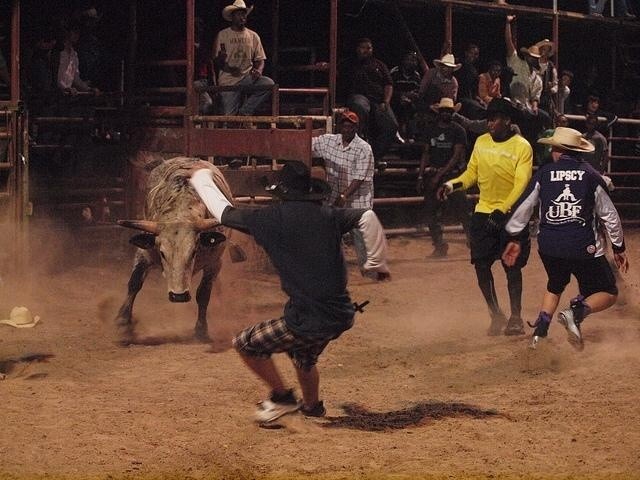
[429,164,465,175]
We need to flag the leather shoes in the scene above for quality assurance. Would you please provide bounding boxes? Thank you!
[375,161,388,168]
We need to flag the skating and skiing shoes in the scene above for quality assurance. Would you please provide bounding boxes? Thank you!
[527,335,544,350]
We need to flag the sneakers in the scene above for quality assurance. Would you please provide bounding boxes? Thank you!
[243,121,258,130]
[425,244,448,258]
[254,388,299,423]
[296,398,326,417]
[556,309,583,350]
[504,316,525,334]
[487,313,508,336]
[229,156,243,167]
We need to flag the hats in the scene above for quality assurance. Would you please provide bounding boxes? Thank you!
[433,54,462,73]
[0,306,39,328]
[340,112,359,124]
[262,162,331,201]
[536,38,554,57]
[520,46,545,59]
[536,127,596,152]
[429,97,461,117]
[222,0,255,22]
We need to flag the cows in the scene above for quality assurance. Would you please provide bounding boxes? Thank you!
[113,155,237,346]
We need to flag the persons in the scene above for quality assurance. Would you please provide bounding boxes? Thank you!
[501,127,630,353]
[190,160,391,424]
[1,1,631,224]
[437,98,533,336]
